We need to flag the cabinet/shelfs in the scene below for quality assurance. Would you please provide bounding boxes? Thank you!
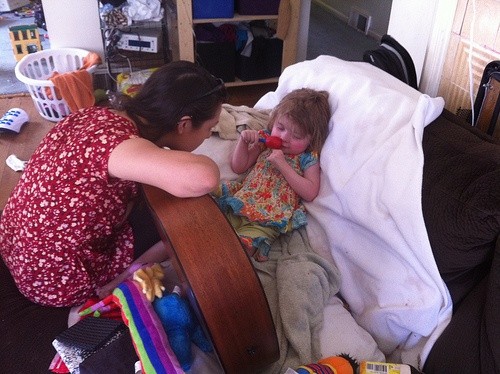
[165,0,303,87]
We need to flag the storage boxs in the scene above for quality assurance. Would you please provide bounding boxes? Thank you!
[15,48,98,123]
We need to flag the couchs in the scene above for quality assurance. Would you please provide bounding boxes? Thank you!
[232,55,500,374]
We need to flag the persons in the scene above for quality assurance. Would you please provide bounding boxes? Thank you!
[0,60,227,344]
[93,88,331,301]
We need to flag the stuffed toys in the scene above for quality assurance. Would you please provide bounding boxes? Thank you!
[78,261,214,373]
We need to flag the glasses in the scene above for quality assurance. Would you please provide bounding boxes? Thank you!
[195,74,224,102]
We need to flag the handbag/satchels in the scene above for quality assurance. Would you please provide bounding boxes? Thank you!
[364,33,418,92]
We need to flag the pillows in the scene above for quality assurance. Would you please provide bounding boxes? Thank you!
[422,108,500,288]
